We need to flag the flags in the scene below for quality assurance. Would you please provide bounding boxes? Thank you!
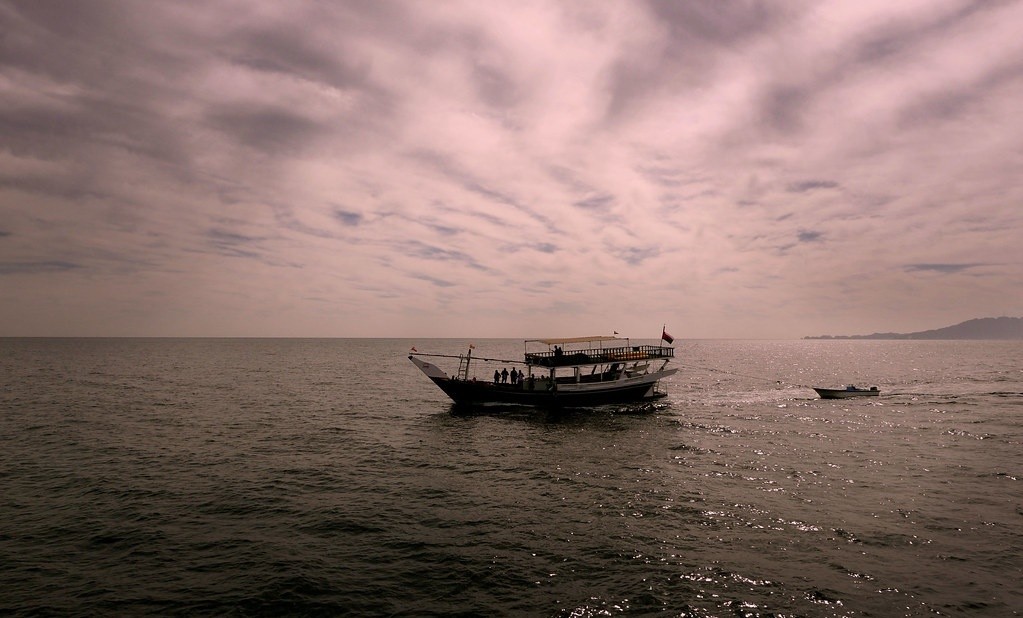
[614,331,618,334]
[470,345,475,349]
[662,332,673,344]
[411,347,417,352]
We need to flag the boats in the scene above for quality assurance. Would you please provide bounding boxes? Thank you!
[813,386,881,400]
[407,348,678,408]
[522,337,674,366]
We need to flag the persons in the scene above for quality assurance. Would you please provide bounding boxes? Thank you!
[608,363,627,381]
[501,368,509,383]
[550,345,563,356]
[510,367,518,384]
[518,370,524,382]
[494,370,501,383]
